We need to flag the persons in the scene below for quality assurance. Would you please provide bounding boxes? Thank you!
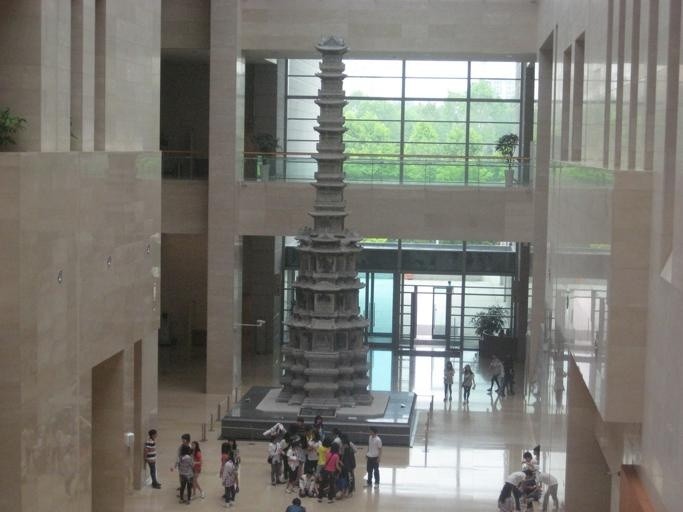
[460,365,475,403]
[171,432,239,506]
[441,360,455,400]
[532,469,559,511]
[497,469,531,511]
[503,352,515,395]
[486,354,504,391]
[142,428,162,488]
[260,414,383,502]
[520,452,537,502]
[284,497,306,511]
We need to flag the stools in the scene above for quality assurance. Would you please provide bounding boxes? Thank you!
[531,499,541,512]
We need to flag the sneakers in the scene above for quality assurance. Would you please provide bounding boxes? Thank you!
[151,481,162,489]
[269,478,380,504]
[174,486,242,507]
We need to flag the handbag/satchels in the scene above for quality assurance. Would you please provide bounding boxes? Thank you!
[267,456,273,464]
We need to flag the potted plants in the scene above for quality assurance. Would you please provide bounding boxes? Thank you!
[257,135,281,184]
[494,132,518,186]
[469,305,509,358]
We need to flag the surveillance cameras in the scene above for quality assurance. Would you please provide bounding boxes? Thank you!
[257,319,266,324]
